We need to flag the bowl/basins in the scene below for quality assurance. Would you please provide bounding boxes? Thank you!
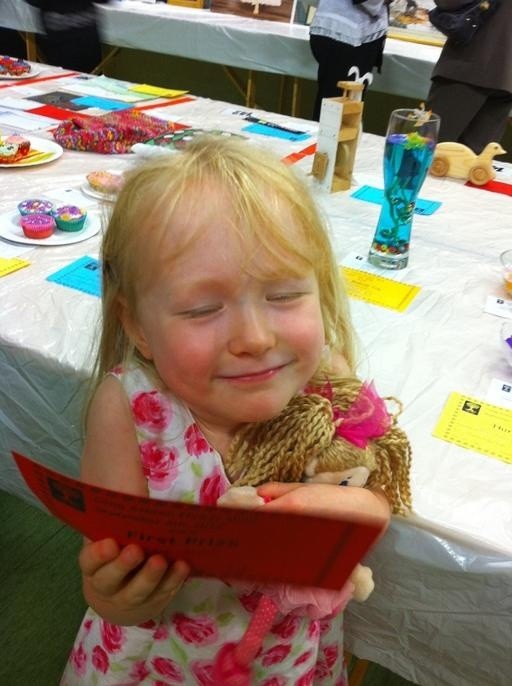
[500,249,512,296]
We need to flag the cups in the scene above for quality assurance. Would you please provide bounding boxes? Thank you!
[368,110,441,269]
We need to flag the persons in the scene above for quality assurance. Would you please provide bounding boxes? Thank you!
[199,374,415,686]
[18,0,118,76]
[422,0,511,160]
[60,129,391,686]
[304,0,390,125]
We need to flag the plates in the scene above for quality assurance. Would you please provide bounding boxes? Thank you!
[81,174,120,202]
[0,209,101,246]
[0,61,40,79]
[1,136,64,168]
